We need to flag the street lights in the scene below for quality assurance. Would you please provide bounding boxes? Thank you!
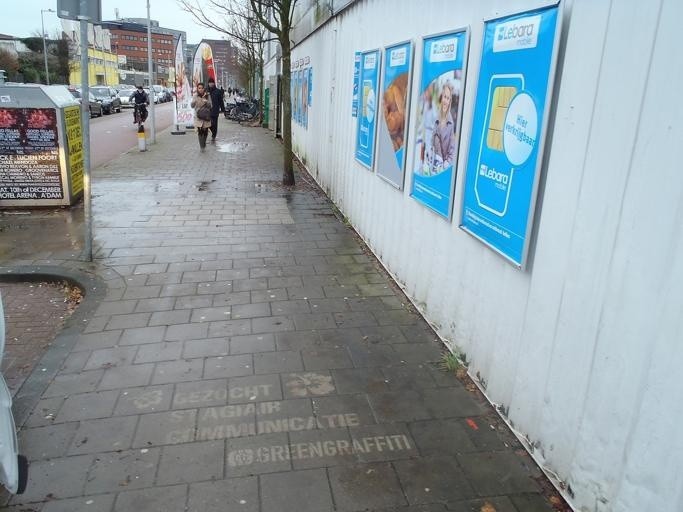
[41,9,57,86]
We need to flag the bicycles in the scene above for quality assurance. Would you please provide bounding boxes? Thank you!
[133,102,144,127]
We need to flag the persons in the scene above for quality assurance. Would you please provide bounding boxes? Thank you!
[220,86,224,98]
[128,86,150,124]
[429,82,456,173]
[228,88,232,97]
[203,78,226,143]
[190,82,214,154]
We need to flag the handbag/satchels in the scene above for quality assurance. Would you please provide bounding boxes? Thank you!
[197,103,210,120]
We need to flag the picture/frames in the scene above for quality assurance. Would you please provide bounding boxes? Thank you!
[355,49,382,170]
[407,25,471,221]
[375,40,415,191]
[459,3,563,275]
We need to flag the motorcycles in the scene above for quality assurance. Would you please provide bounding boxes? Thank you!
[224,97,265,124]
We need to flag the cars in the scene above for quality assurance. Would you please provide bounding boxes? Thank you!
[69,84,173,117]
